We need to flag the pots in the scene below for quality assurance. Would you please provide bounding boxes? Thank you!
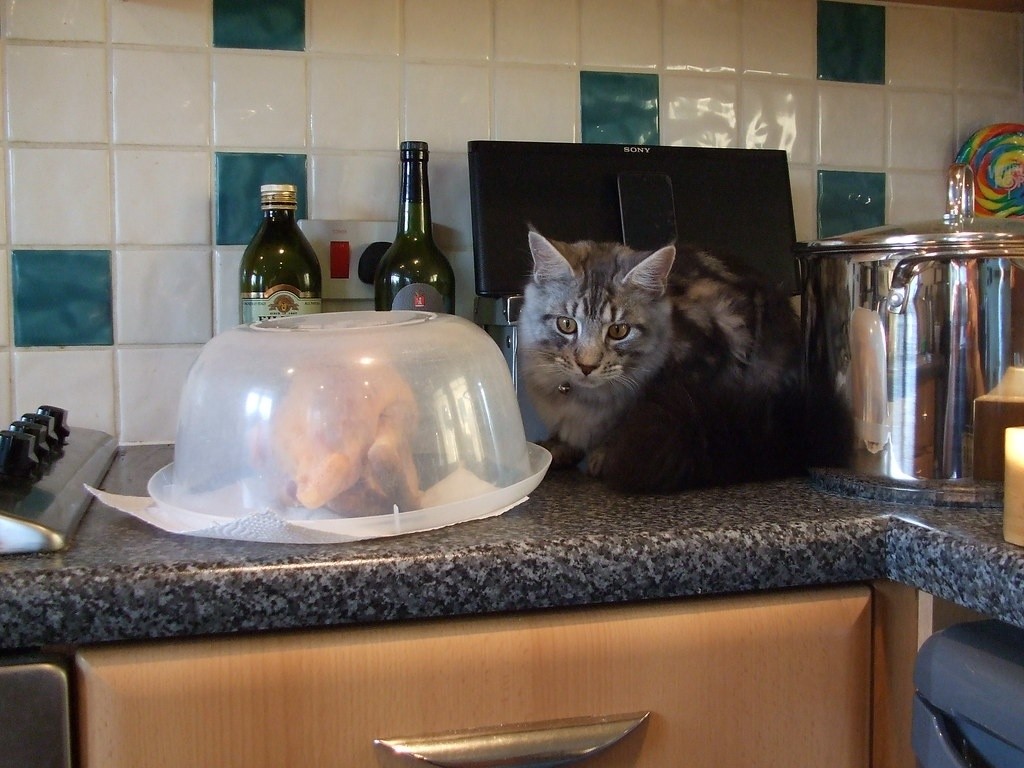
[788,163,1023,506]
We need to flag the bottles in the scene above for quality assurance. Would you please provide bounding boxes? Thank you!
[239,184,323,325]
[375,141,457,314]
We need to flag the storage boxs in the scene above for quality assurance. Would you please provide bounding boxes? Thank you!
[147,310,552,541]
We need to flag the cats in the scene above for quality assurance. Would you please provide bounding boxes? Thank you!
[515,230,805,495]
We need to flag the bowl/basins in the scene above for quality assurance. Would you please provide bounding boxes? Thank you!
[145,308,554,535]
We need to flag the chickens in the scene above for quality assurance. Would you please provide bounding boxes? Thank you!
[272,352,422,518]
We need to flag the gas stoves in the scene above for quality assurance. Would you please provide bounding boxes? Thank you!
[0,404,120,554]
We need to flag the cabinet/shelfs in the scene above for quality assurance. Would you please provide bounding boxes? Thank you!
[75,585,911,768]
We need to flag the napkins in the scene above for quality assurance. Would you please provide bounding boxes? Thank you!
[81,482,531,545]
